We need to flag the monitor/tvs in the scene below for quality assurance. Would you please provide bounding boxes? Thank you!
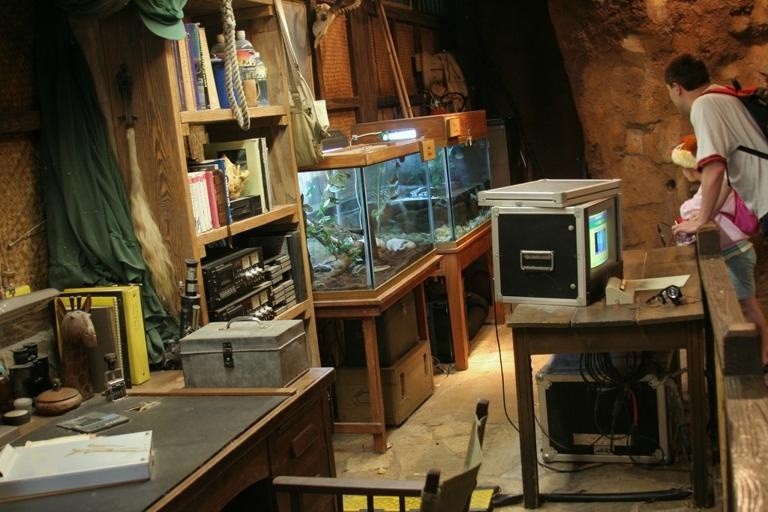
[491,192,628,308]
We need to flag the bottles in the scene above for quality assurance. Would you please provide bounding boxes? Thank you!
[210,30,269,107]
[8,342,51,402]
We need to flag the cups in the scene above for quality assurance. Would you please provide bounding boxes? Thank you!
[672,227,689,247]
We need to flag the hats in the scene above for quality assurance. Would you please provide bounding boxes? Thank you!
[670,138,698,169]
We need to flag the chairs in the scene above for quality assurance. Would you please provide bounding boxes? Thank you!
[271,399,489,512]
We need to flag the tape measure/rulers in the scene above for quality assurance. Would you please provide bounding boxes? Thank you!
[126,388,296,396]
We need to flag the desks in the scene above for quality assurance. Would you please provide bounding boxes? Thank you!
[1,367,342,512]
[505,245,716,507]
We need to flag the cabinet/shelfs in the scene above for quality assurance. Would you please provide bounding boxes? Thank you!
[100,1,321,369]
[265,390,341,512]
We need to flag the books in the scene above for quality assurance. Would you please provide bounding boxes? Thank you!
[50,280,150,386]
[188,135,271,232]
[0,429,156,502]
[173,15,222,112]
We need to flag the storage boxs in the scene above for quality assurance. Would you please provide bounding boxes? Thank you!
[178,316,308,388]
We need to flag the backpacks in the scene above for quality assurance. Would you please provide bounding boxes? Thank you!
[733,193,758,233]
[699,80,767,161]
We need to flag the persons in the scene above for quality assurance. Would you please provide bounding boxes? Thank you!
[670,134,767,369]
[666,54,767,310]
